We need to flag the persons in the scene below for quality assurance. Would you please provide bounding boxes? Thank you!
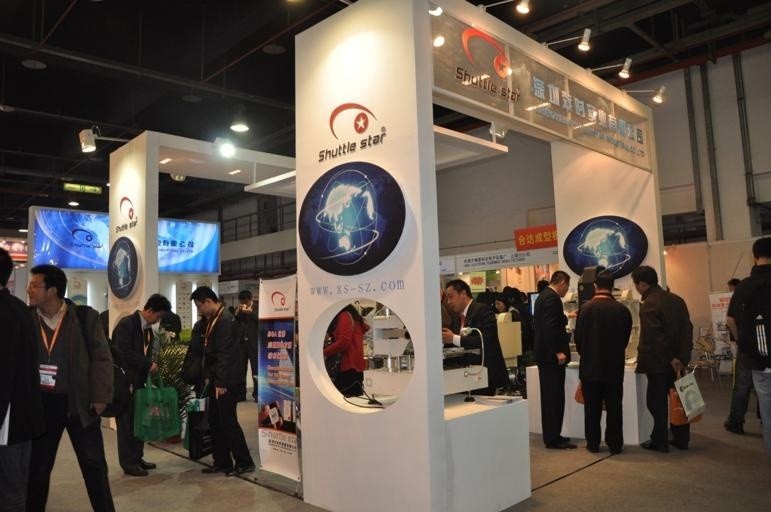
[439,266,694,455]
[0,244,49,512]
[323,305,370,397]
[723,236,771,455]
[105,286,258,478]
[27,263,120,512]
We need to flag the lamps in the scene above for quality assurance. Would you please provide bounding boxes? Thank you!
[542,27,592,52]
[458,325,486,378]
[477,0,533,16]
[76,122,130,154]
[621,86,666,104]
[587,57,632,80]
[228,103,249,133]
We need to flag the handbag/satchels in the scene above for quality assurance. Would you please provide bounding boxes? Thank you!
[323,338,340,381]
[184,384,212,461]
[134,371,181,441]
[100,338,130,417]
[669,367,706,425]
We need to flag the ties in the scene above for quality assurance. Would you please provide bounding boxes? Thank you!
[460,314,466,328]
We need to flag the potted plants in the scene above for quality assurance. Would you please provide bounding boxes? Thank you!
[145,332,195,444]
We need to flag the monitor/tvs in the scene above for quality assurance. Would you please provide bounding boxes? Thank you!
[527,291,541,317]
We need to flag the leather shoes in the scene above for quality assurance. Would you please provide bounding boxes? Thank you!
[543,434,688,453]
[140,461,156,469]
[122,465,148,476]
[227,464,255,476]
[202,464,233,473]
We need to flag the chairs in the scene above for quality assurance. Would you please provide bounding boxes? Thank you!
[691,335,734,392]
